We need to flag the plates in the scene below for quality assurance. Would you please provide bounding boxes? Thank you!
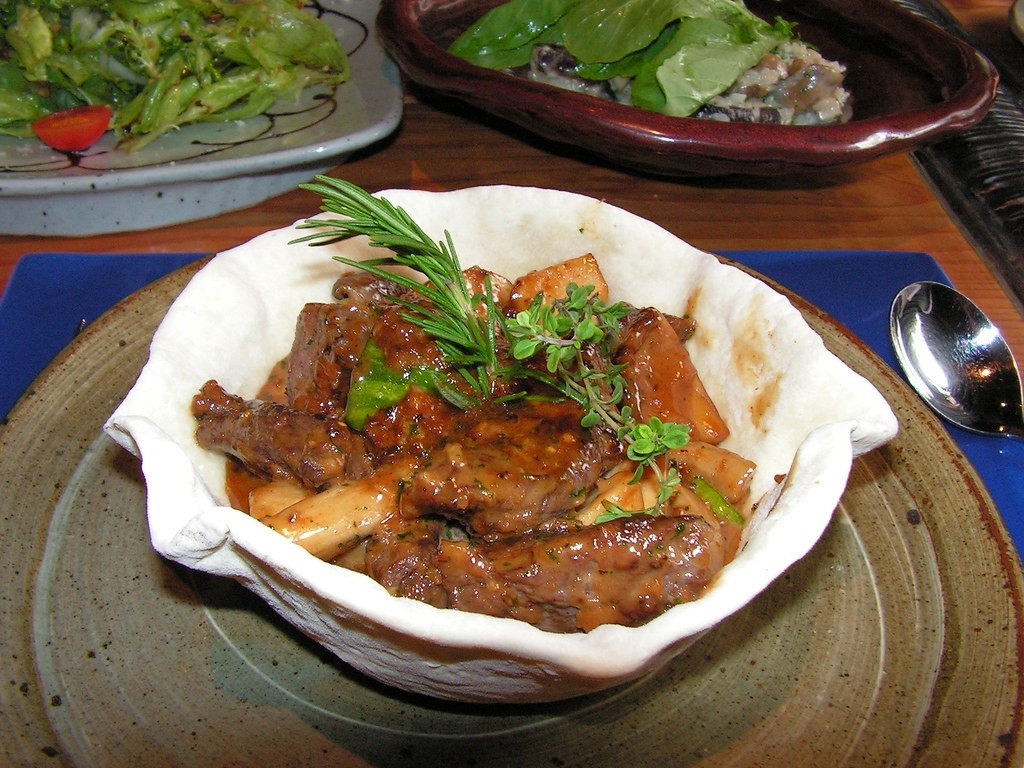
[375,0,1000,182]
[0,0,404,237]
[0,253,1024,768]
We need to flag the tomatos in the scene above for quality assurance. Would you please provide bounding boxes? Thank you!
[33,105,111,151]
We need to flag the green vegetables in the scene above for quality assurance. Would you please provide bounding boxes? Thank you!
[286,173,690,524]
[0,0,350,151]
[446,0,798,118]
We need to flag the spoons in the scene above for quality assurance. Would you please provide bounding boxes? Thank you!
[889,281,1024,443]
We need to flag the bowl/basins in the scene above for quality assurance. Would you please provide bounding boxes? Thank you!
[103,185,900,703]
